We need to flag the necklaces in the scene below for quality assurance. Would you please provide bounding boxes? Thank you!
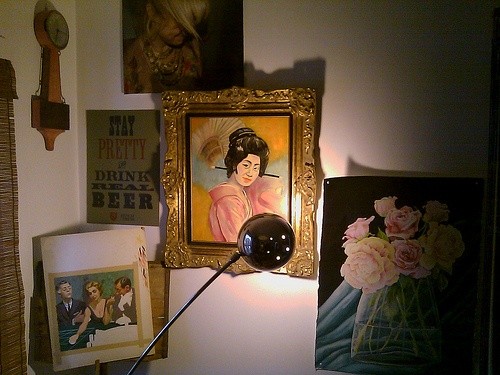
[144,39,183,91]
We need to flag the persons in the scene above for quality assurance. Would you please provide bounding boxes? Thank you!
[123,0,209,94]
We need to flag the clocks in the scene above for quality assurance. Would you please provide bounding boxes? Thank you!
[31,9,70,151]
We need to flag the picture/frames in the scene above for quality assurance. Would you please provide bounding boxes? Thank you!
[163,85,317,278]
